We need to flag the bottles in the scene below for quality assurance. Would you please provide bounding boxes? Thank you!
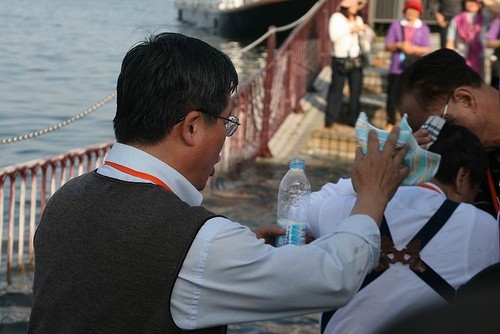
[276,158,311,246]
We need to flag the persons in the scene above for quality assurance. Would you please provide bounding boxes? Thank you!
[393,47,499,226]
[30,32,410,334]
[447,1,485,84]
[385,0,430,127]
[324,0,376,127]
[290,124,500,333]
[479,0,500,91]
[425,1,464,51]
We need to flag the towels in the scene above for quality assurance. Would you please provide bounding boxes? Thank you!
[353,111,446,187]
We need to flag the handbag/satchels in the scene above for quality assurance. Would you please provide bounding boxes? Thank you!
[398,50,421,70]
[333,50,368,74]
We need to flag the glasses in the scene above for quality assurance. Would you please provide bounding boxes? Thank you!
[172,109,241,137]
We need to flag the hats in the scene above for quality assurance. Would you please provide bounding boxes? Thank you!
[337,0,367,12]
[404,0,424,15]
